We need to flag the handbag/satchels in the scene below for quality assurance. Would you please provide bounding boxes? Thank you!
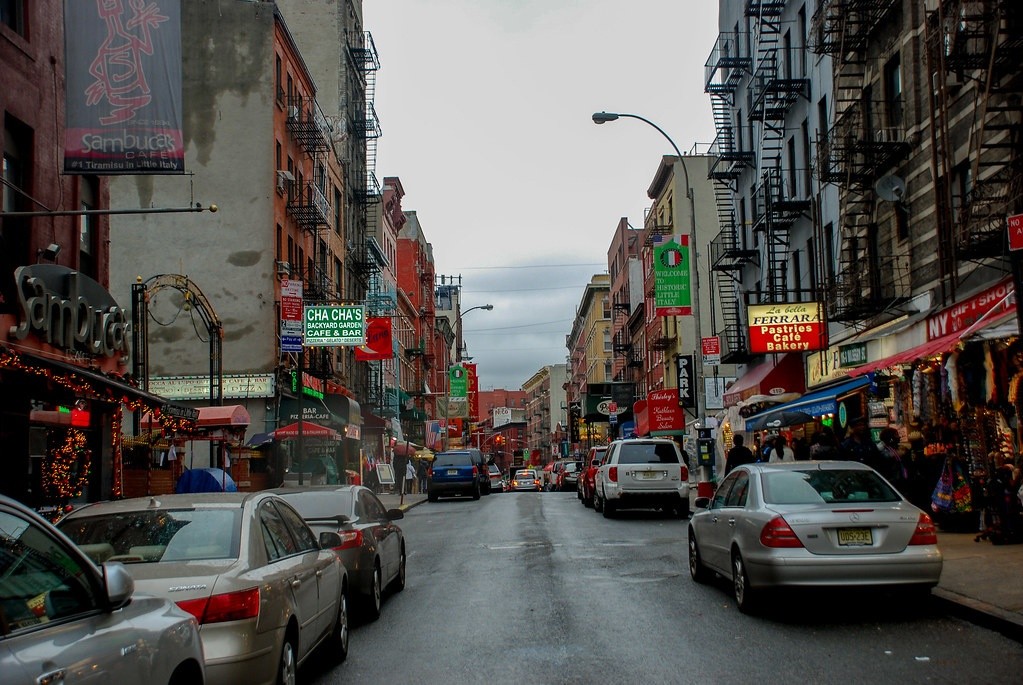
[930,459,977,516]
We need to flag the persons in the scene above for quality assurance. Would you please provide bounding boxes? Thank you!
[392,455,409,495]
[417,458,429,494]
[259,439,289,487]
[761,416,914,497]
[406,461,416,493]
[724,434,755,477]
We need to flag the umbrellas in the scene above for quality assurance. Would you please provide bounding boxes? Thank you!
[393,443,415,454]
[752,411,816,431]
[274,420,336,437]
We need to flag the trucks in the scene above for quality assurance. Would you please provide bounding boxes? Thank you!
[509,466,527,482]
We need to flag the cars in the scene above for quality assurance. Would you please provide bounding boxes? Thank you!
[688,460,942,614]
[511,469,541,492]
[575,444,610,508]
[446,448,491,496]
[0,492,350,685]
[263,485,406,620]
[486,463,503,493]
[549,461,587,492]
[0,494,206,685]
[421,452,481,502]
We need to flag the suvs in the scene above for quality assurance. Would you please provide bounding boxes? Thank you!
[591,438,689,518]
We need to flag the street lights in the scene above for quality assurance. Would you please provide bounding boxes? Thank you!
[445,304,493,450]
[592,112,718,495]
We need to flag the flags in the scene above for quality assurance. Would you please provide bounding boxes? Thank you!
[426,422,438,446]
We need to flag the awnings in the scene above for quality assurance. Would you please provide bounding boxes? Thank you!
[724,353,804,407]
[288,400,345,426]
[138,405,251,490]
[20,353,199,420]
[747,377,872,432]
[847,307,1016,377]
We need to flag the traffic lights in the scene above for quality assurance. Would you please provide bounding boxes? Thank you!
[499,436,505,446]
